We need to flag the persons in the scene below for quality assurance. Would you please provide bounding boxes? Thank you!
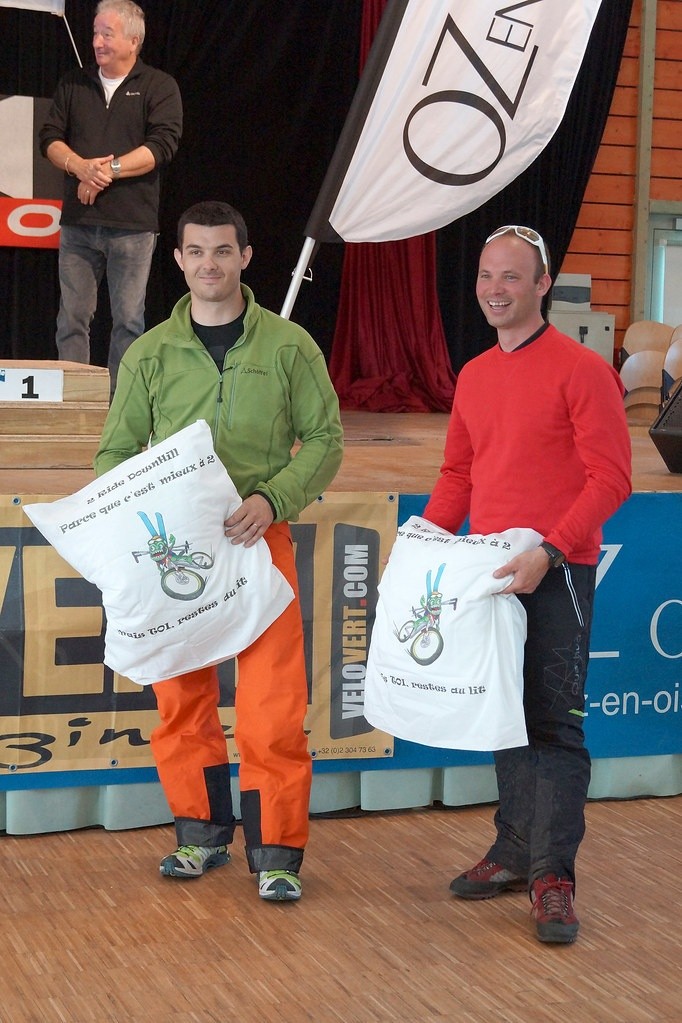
[422,223,631,950]
[37,1,183,411]
[87,201,343,907]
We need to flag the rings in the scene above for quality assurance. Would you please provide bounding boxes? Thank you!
[86,190,91,194]
[252,523,259,528]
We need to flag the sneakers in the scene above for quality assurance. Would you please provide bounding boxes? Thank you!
[529,873,580,943]
[159,843,231,878]
[448,856,528,900]
[254,869,302,901]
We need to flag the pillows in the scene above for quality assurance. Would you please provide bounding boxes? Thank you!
[360,516,548,760]
[15,414,299,686]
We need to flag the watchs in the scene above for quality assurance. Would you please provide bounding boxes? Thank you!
[110,159,121,179]
[539,543,567,569]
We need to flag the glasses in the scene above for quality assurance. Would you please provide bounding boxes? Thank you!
[485,225,549,276]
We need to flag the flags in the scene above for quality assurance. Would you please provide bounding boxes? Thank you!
[330,1,633,381]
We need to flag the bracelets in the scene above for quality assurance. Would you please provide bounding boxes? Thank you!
[65,153,77,176]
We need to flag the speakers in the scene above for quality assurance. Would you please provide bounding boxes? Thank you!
[649,380,682,474]
